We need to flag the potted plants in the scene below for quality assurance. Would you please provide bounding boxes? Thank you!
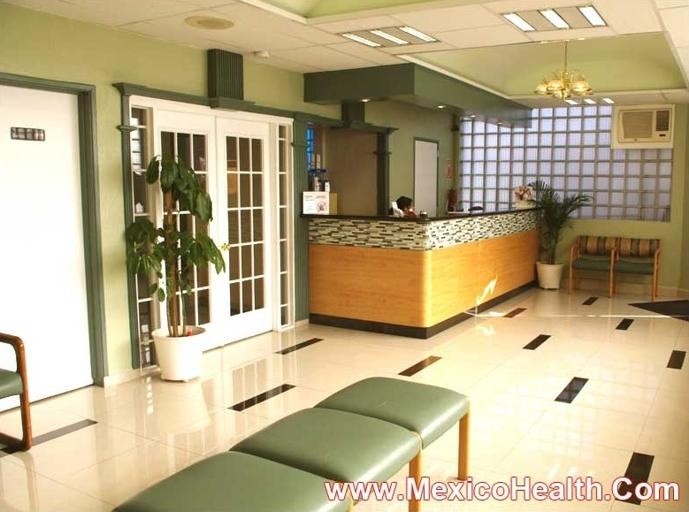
[126,154,226,381]
[526,179,594,289]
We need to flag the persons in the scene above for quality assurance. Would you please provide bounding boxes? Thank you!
[386,196,418,219]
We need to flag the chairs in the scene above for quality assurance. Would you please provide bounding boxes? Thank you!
[571,236,615,297]
[0,332,32,452]
[613,236,661,299]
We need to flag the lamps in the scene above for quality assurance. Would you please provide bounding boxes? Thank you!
[534,41,592,100]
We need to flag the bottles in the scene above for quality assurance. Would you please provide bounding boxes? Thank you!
[314,177,319,191]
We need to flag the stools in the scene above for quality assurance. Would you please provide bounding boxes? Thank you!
[314,377,470,512]
[229,408,422,511]
[111,450,353,512]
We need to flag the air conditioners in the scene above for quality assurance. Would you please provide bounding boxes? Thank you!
[618,107,672,143]
